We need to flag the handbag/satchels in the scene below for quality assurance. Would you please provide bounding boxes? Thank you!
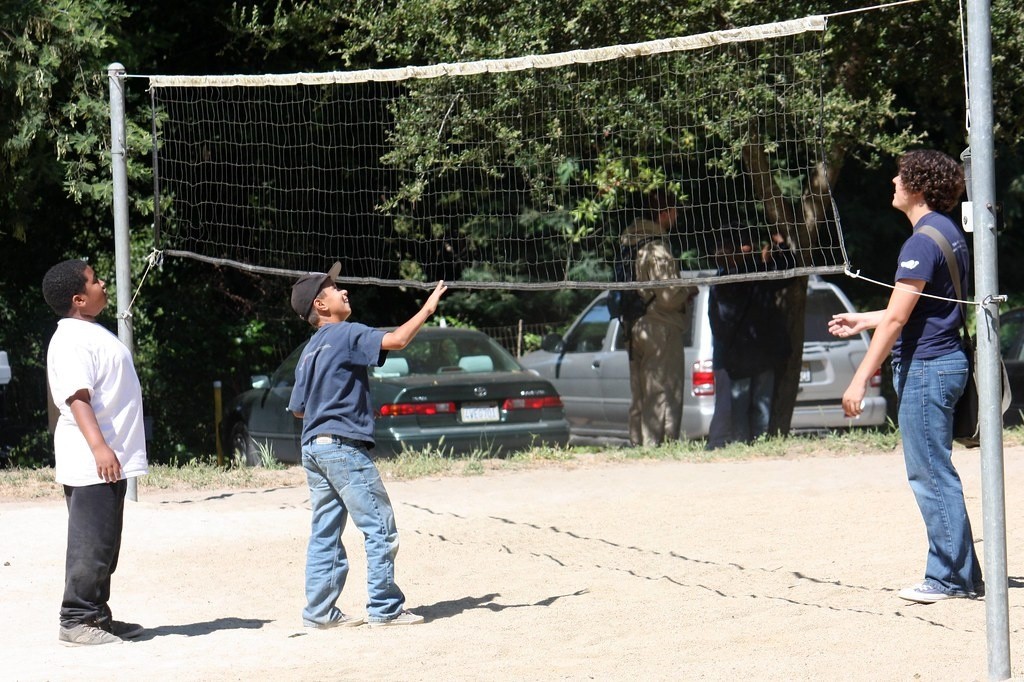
[952,340,980,449]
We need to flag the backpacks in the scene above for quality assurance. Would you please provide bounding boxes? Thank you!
[606,237,662,319]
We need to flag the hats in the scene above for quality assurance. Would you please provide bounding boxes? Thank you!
[291,260,342,320]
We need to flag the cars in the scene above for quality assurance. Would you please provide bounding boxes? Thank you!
[219,327,571,470]
[971,307,1024,426]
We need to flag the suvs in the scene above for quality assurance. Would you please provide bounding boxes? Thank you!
[519,268,887,448]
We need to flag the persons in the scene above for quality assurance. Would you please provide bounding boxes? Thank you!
[704,228,813,452]
[827,149,985,605]
[40,258,150,646]
[288,272,450,627]
[617,187,699,449]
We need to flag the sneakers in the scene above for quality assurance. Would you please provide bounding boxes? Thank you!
[898,583,966,602]
[330,613,364,627]
[368,610,425,628]
[93,619,143,639]
[58,620,122,647]
